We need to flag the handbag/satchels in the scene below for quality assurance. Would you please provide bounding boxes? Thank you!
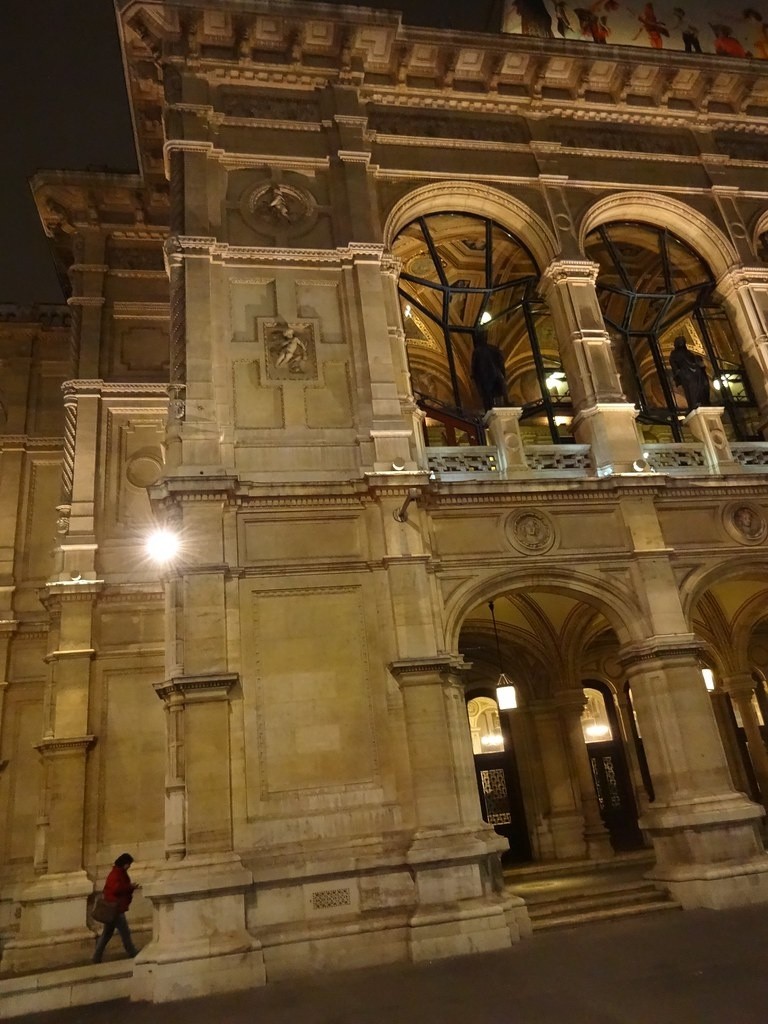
[92,900,119,923]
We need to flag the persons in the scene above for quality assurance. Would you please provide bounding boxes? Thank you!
[470,334,513,409]
[92,851,142,962]
[669,336,711,411]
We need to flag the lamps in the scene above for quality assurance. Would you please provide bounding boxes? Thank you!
[489,601,518,710]
